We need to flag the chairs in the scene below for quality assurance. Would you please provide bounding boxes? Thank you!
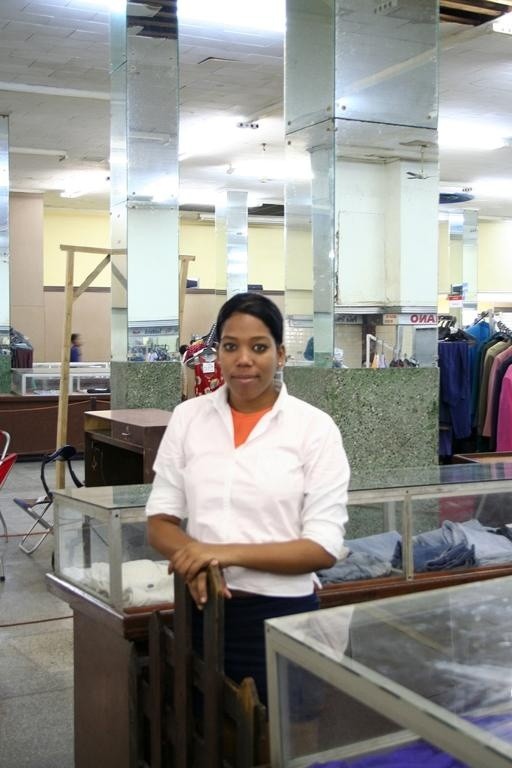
[12,446,110,553]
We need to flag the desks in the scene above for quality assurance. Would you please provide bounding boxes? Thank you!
[85,406,175,485]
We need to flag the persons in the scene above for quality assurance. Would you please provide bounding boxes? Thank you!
[70,334,84,362]
[145,293,351,768]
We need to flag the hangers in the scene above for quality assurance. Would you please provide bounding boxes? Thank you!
[439,316,477,343]
